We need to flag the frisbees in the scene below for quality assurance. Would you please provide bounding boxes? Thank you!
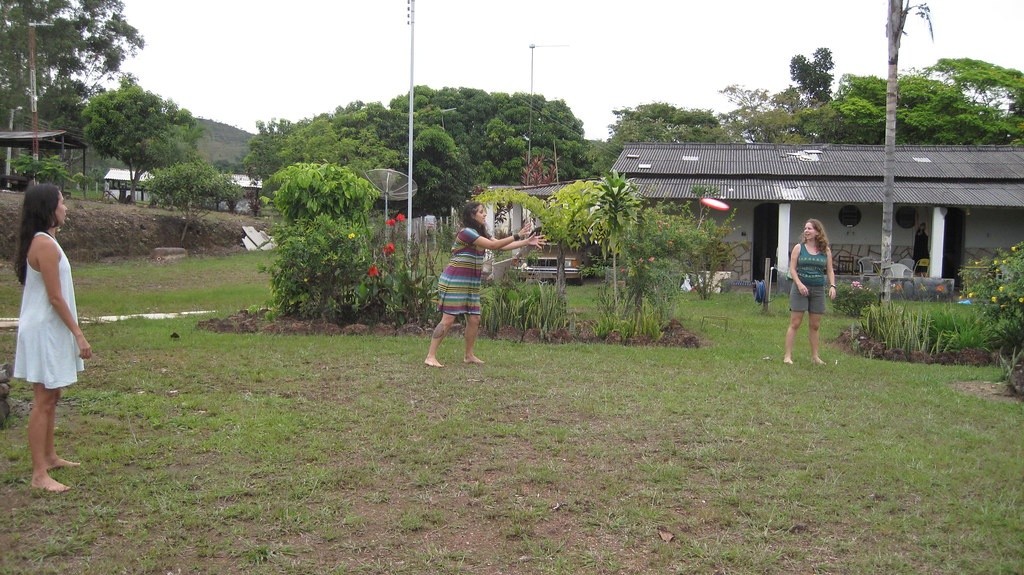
[701,197,729,209]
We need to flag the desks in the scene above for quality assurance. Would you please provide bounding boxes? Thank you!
[966,265,990,292]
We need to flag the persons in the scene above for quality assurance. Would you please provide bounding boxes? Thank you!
[912,221,928,277]
[16,184,94,494]
[783,219,837,366]
[423,202,548,368]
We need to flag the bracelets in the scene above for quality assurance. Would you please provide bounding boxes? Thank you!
[513,234,519,240]
[830,285,837,289]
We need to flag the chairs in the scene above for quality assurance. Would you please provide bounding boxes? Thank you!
[839,255,854,275]
[858,256,930,277]
